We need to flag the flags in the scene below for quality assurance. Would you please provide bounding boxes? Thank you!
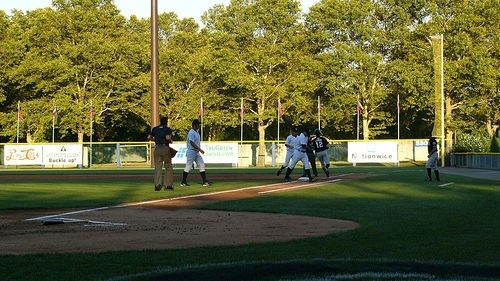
[240,102,245,117]
[278,100,282,118]
[356,99,364,112]
[89,102,93,119]
[200,98,204,116]
[53,105,57,119]
[16,104,23,120]
[397,96,400,113]
[318,97,323,116]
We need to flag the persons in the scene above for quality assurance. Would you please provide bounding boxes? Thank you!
[426,130,441,182]
[152,117,174,191]
[180,119,214,187]
[276,125,318,181]
[313,130,330,177]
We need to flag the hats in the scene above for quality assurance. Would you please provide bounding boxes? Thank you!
[315,130,321,134]
[291,126,299,132]
[303,128,309,132]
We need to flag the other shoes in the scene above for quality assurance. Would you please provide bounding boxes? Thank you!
[154,184,162,191]
[164,186,174,191]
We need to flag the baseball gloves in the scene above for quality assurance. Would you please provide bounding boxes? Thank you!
[169,147,178,158]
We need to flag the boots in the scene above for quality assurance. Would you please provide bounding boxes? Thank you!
[301,171,307,177]
[434,170,440,181]
[180,172,189,186]
[277,165,284,176]
[305,169,314,181]
[426,168,432,181]
[322,167,329,176]
[201,171,211,186]
[326,163,330,168]
[313,167,318,176]
[285,167,293,181]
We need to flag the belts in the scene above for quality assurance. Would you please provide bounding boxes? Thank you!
[298,149,306,153]
